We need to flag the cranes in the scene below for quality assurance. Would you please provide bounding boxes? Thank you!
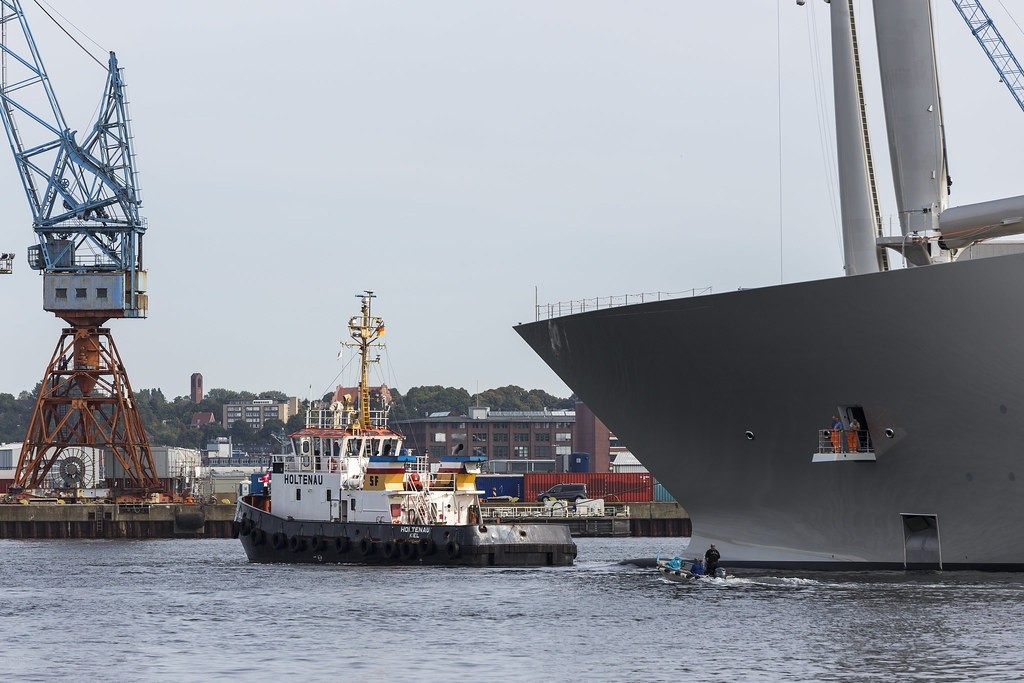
[0,1,198,503]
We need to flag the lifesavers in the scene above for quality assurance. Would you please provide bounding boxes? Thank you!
[334,536,346,553]
[445,541,461,560]
[382,540,396,558]
[358,537,371,556]
[230,521,240,539]
[416,537,435,557]
[238,519,250,536]
[310,534,322,553]
[270,532,286,551]
[398,541,416,560]
[250,527,263,547]
[287,535,302,553]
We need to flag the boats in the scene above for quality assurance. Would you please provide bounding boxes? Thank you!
[655,556,734,584]
[514,0,1024,579]
[233,289,578,570]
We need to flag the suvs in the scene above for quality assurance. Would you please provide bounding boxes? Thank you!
[536,481,588,503]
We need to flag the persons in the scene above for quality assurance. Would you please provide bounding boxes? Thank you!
[847,419,860,453]
[325,438,391,456]
[705,544,720,575]
[831,415,842,453]
[62,355,68,370]
[671,557,680,568]
[690,556,704,575]
